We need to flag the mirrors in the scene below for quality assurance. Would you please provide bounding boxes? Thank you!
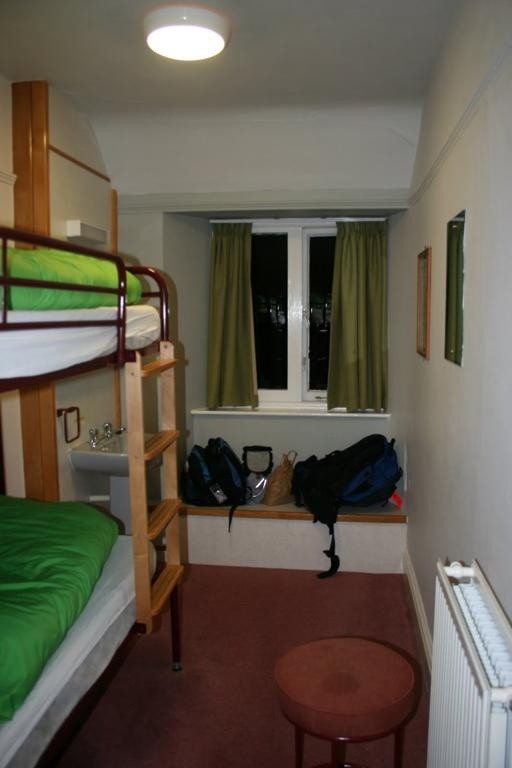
[64,407,81,444]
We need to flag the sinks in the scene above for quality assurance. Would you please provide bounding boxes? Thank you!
[72,432,155,475]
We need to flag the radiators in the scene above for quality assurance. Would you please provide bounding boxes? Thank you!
[421,554,509,766]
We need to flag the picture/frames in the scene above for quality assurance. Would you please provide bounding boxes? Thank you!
[414,239,432,363]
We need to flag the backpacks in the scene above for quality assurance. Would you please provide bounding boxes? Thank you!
[291,434,404,526]
[178,437,251,508]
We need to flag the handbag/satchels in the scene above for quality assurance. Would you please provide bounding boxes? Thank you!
[241,444,274,505]
[263,450,298,507]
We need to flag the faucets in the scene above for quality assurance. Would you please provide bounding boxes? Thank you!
[103,422,125,440]
[89,426,112,446]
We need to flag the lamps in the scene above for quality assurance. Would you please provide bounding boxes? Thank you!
[142,3,233,65]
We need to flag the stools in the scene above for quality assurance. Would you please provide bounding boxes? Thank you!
[273,635,420,762]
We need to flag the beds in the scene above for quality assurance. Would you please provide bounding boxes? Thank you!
[1,222,186,761]
